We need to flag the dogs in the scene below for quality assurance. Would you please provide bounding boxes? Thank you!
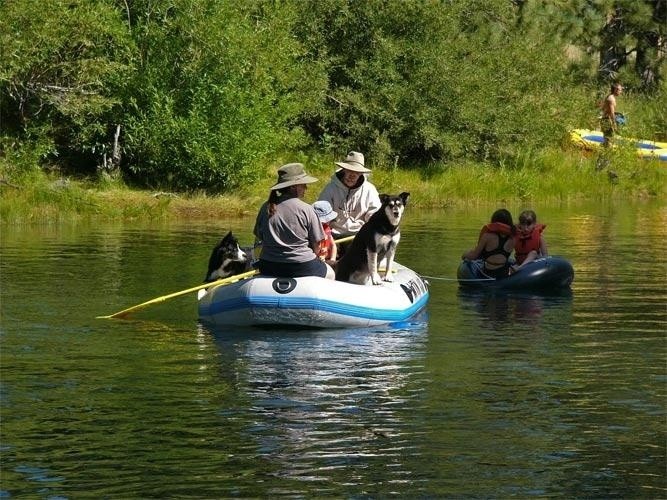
[197,231,260,302]
[334,192,410,285]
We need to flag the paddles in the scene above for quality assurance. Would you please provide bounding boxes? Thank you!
[111,234,355,317]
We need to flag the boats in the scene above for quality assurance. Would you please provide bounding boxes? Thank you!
[196,253,429,330]
[566,127,666,161]
[456,258,573,296]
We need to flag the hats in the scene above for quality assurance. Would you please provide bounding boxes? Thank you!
[310,200,339,225]
[268,161,320,191]
[334,150,373,174]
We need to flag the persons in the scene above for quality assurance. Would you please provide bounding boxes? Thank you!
[253,161,336,280]
[509,209,547,272]
[319,151,383,254]
[460,208,516,280]
[311,199,336,262]
[594,83,623,170]
